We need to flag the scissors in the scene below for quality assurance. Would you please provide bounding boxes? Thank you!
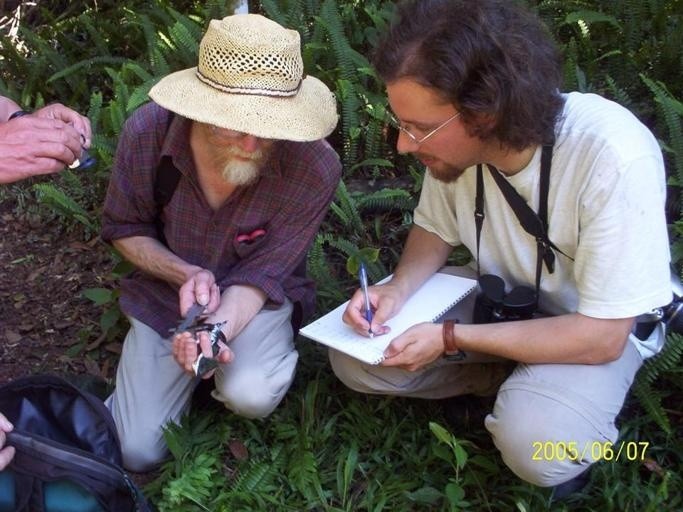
[238,230,267,244]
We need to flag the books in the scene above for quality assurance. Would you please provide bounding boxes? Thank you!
[298,273,477,366]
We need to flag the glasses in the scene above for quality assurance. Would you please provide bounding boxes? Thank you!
[388,107,462,146]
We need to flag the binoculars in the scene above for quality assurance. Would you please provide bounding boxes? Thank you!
[473,272,539,324]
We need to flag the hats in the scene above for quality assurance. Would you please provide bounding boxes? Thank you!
[148,13,340,143]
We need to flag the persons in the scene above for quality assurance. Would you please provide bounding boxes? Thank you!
[325,1,674,501]
[1,96,94,186]
[106,15,343,472]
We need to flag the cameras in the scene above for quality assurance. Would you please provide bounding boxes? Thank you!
[65,121,96,172]
[632,271,683,342]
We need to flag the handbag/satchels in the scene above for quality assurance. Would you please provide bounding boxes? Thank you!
[0,371,155,512]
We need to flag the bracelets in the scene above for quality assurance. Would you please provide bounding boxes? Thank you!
[443,319,465,362]
[8,110,28,121]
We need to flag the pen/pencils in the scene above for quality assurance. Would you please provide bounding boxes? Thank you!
[359,262,374,338]
